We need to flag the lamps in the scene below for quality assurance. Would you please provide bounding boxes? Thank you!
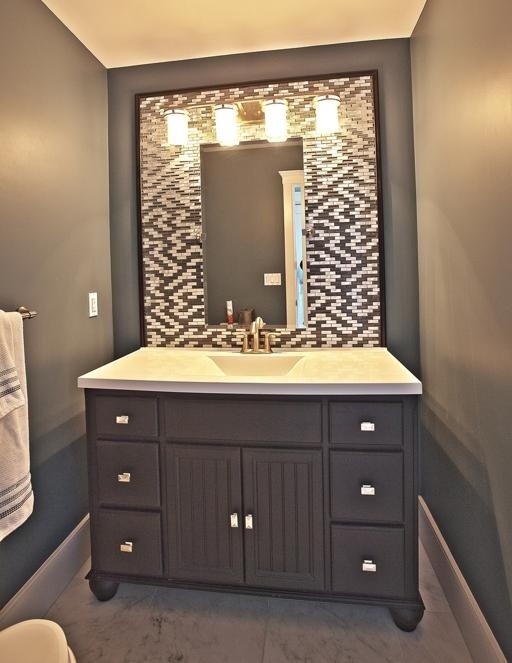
[164,87,343,146]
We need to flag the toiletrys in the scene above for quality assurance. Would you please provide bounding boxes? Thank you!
[226,299,234,329]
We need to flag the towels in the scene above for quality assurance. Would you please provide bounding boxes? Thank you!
[0,308,35,539]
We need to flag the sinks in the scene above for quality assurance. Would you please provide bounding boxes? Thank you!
[190,352,306,382]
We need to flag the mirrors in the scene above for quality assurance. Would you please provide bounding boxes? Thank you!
[200,135,307,329]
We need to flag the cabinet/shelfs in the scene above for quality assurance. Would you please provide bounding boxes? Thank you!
[329,394,421,608]
[164,391,328,443]
[165,445,325,599]
[85,387,162,581]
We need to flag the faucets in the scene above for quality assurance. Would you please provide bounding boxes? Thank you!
[250,317,266,351]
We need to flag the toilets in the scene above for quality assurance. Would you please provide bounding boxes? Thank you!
[0,617,76,662]
